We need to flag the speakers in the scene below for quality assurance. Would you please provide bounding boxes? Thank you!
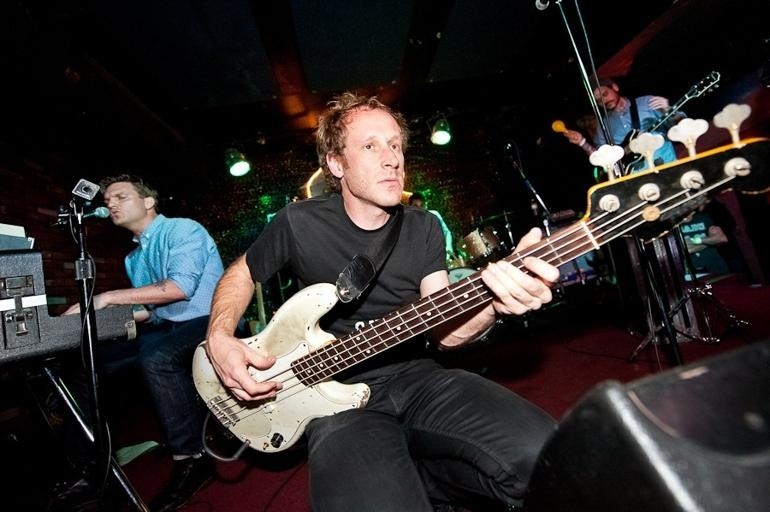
[522,341,769,511]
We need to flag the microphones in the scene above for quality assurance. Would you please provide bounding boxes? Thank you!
[83,206,111,219]
[504,143,513,154]
[535,0,550,11]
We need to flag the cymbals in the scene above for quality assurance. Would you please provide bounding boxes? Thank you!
[472,212,510,226]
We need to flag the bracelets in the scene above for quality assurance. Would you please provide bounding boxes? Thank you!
[579,137,586,147]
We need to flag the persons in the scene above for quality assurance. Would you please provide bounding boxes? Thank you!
[564,78,687,171]
[679,211,730,274]
[206,92,559,512]
[409,192,454,261]
[697,197,758,286]
[59,174,246,512]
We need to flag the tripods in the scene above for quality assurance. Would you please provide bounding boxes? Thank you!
[625,222,758,363]
[47,226,140,512]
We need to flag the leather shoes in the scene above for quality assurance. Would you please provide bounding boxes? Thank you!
[149,461,214,512]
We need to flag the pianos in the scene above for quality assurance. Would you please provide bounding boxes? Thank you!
[1,252,138,364]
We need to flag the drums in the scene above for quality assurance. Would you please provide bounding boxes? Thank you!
[447,258,478,283]
[455,224,506,266]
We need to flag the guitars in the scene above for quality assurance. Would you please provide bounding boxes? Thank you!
[594,71,721,184]
[193,105,768,463]
